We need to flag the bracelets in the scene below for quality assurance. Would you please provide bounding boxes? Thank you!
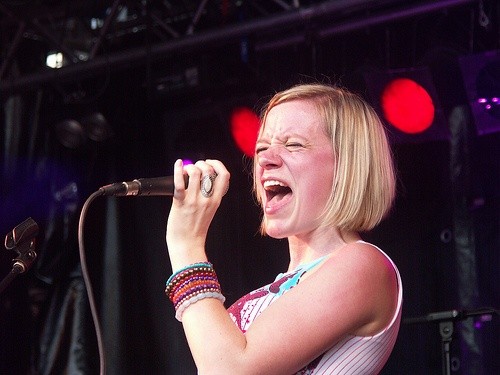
[165,262,226,321]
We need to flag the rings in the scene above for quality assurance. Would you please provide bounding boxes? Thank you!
[201,175,214,198]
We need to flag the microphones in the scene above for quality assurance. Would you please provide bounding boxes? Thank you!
[100,171,229,196]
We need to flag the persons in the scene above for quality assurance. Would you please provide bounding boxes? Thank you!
[167,84,404,375]
